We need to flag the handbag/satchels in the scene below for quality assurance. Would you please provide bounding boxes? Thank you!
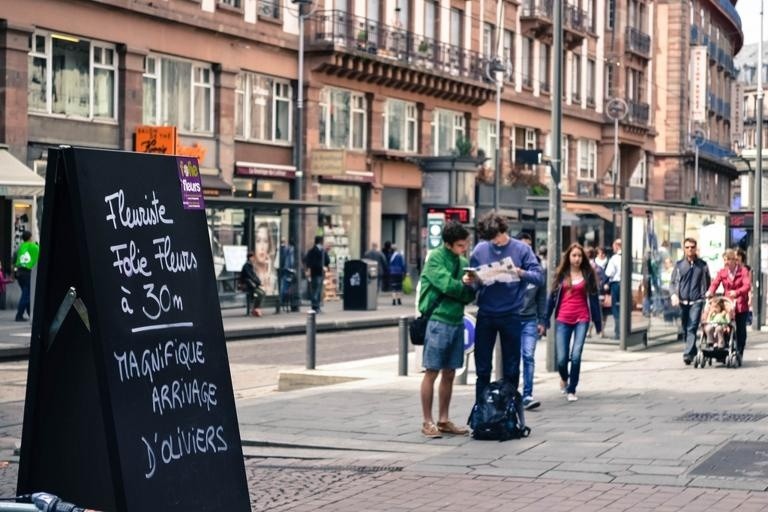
[237,283,247,290]
[602,294,612,309]
[408,316,427,346]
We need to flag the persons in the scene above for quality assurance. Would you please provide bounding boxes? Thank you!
[705,299,725,349]
[738,251,752,278]
[466,213,545,406]
[510,233,546,409]
[418,221,473,437]
[237,224,410,317]
[541,243,602,401]
[583,249,607,338]
[598,248,609,269]
[606,240,628,339]
[11,232,36,322]
[705,248,750,366]
[670,239,710,365]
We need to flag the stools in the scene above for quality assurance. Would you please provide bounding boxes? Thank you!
[242,289,254,314]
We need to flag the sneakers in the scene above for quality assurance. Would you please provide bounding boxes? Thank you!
[421,421,443,439]
[436,420,469,435]
[522,395,541,410]
[251,308,263,317]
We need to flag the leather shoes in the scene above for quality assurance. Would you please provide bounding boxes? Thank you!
[682,356,692,366]
[567,392,578,402]
[558,378,568,395]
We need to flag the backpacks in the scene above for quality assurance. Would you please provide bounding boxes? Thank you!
[467,379,532,442]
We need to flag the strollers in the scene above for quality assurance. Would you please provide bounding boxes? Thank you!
[695,293,741,368]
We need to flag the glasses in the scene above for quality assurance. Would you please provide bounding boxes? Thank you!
[685,246,696,248]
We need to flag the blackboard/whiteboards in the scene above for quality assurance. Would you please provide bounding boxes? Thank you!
[61,145,252,511]
[342,259,366,312]
[361,258,380,310]
